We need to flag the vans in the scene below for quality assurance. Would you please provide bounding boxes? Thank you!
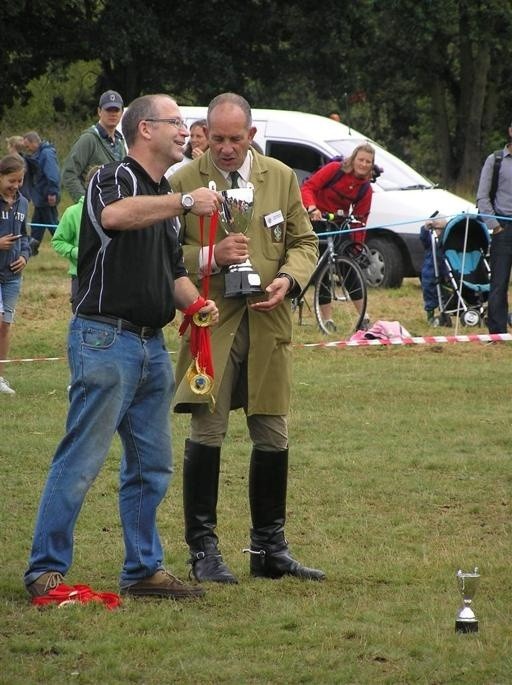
[114,105,481,292]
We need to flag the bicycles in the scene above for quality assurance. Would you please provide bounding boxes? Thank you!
[281,209,369,341]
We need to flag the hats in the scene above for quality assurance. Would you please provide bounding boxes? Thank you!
[97,88,126,110]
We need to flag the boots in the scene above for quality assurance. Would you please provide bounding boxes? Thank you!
[245,444,328,583]
[181,434,242,588]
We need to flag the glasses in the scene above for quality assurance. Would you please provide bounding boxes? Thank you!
[144,116,190,131]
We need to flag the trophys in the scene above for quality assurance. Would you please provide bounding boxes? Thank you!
[208,180,266,298]
[454,566,481,634]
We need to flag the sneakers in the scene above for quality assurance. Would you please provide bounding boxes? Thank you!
[122,565,208,599]
[0,377,17,395]
[25,569,69,604]
[356,318,371,332]
[324,318,338,333]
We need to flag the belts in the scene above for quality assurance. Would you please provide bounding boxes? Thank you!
[75,303,160,340]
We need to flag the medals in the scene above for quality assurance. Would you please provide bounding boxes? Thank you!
[193,309,213,328]
[186,353,217,417]
[56,599,84,609]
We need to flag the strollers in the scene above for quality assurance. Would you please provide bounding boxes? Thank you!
[420,206,492,330]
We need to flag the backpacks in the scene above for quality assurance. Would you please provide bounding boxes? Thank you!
[299,154,373,207]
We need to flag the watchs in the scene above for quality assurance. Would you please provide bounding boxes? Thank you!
[179,191,194,216]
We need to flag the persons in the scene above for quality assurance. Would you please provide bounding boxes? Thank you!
[0,152,33,395]
[49,165,103,314]
[24,94,227,599]
[5,135,32,234]
[301,140,378,332]
[183,118,210,162]
[23,130,62,256]
[476,121,512,334]
[167,92,326,587]
[418,217,448,326]
[62,90,128,203]
